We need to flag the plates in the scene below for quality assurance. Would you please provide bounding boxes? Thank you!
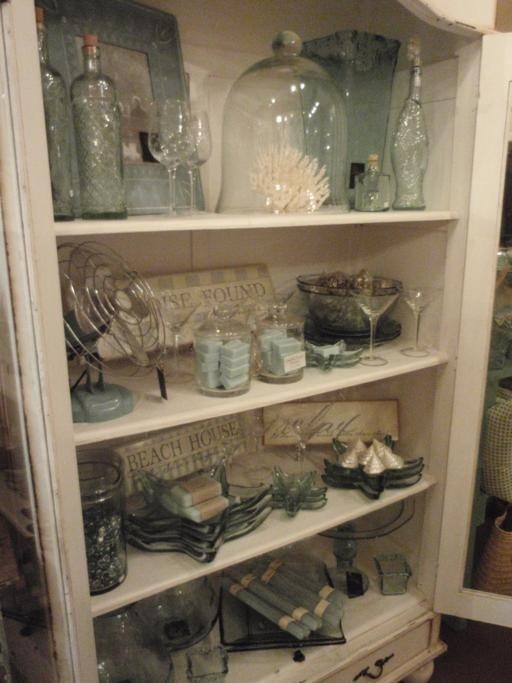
[304,338,364,372]
[259,463,328,517]
[302,315,401,351]
[320,433,425,500]
[125,453,274,564]
[217,559,347,652]
[316,496,416,599]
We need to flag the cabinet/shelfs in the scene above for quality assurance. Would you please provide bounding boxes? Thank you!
[0,0,511,683]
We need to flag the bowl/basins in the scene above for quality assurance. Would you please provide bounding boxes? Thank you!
[295,272,403,335]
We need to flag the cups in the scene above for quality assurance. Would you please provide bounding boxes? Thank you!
[395,283,444,356]
[180,110,212,212]
[159,300,202,384]
[185,644,230,683]
[349,289,402,365]
[372,552,413,595]
[76,448,128,595]
[148,99,193,212]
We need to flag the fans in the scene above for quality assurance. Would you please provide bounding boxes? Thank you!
[48,239,166,430]
